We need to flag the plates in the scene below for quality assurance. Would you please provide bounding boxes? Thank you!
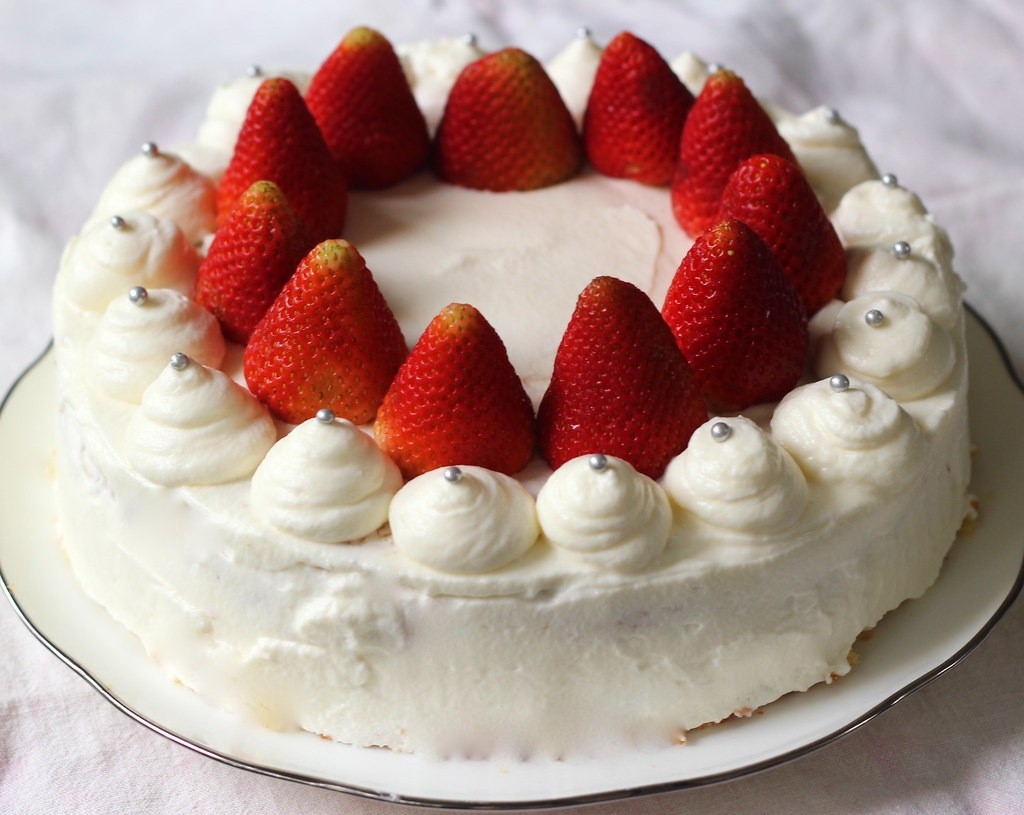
[0,302,1023,807]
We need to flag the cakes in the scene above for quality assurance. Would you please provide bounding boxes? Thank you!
[47,23,973,766]
